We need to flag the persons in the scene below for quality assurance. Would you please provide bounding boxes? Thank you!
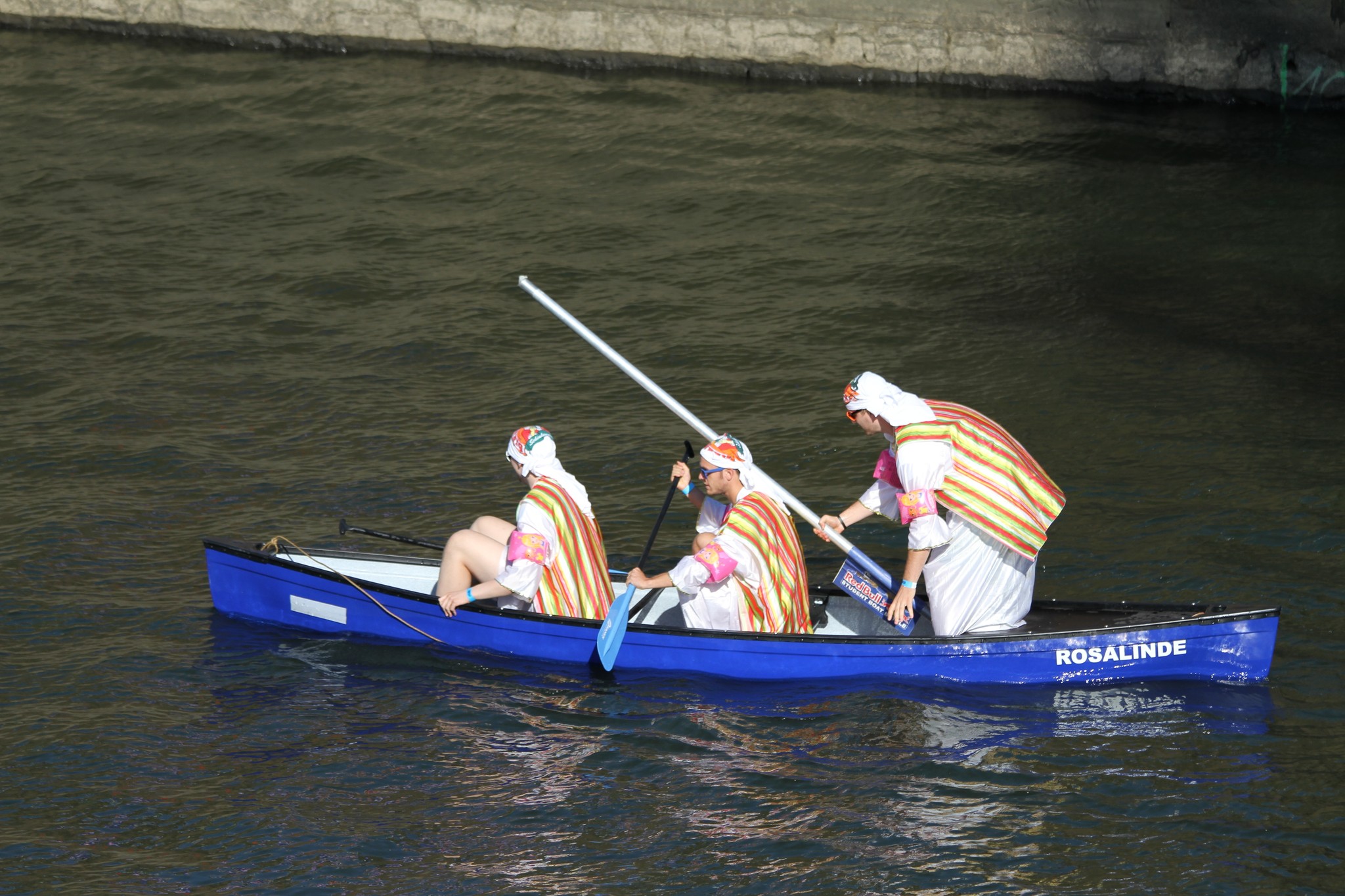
[626,432,814,634]
[813,371,1067,635]
[435,425,616,620]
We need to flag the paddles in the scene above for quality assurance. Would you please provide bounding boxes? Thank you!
[596,438,696,672]
[336,517,445,552]
[516,271,929,615]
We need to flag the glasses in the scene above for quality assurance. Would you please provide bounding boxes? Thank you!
[846,408,863,422]
[700,467,737,479]
[505,451,512,463]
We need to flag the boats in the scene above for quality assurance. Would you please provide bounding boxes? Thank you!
[200,532,1284,687]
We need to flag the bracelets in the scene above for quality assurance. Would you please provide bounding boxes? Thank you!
[681,481,695,495]
[837,515,847,530]
[466,587,476,602]
[901,579,917,589]
